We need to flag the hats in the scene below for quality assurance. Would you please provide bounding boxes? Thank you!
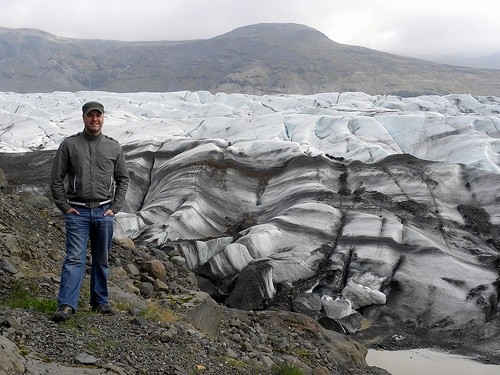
[82,101,104,115]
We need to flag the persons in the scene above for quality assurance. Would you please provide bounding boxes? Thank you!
[51,100,129,321]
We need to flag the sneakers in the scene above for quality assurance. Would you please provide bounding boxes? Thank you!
[53,304,73,321]
[91,303,118,316]
